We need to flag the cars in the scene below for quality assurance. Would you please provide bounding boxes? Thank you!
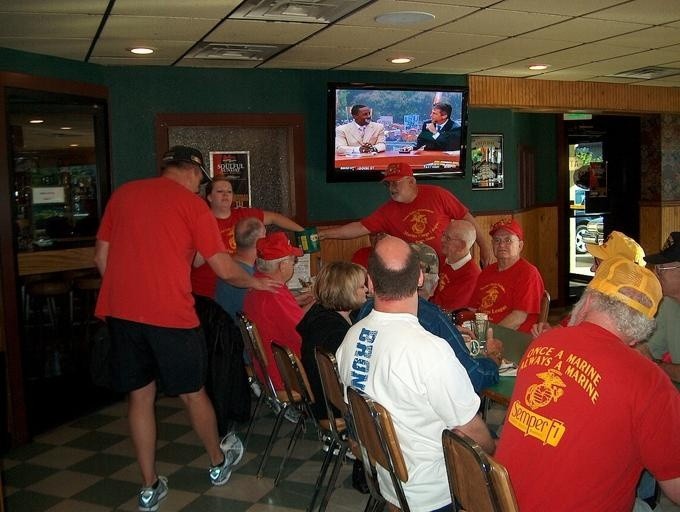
[574,188,602,254]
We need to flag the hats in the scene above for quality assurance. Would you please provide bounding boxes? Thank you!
[585,231,647,268]
[489,220,524,243]
[256,233,304,260]
[161,145,212,185]
[588,258,663,320]
[643,232,680,264]
[379,163,414,183]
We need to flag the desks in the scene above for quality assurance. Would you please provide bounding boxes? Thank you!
[480,320,534,425]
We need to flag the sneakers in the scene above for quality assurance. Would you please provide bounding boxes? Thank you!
[209,431,244,485]
[332,438,356,460]
[321,430,332,451]
[137,476,168,511]
[271,399,300,423]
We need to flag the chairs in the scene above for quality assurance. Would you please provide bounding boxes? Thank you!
[305,345,353,512]
[344,382,412,512]
[439,428,522,512]
[537,290,550,321]
[233,313,306,478]
[269,339,315,490]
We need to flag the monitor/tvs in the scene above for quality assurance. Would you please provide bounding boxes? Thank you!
[326,81,469,184]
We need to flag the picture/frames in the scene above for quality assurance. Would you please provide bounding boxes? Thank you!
[471,134,504,191]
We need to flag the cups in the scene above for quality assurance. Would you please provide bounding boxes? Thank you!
[461,332,480,358]
[475,313,488,323]
[470,319,490,342]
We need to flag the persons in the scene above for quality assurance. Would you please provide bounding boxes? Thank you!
[466,219,544,334]
[530,231,671,362]
[95,145,285,512]
[401,103,462,153]
[243,232,318,390]
[296,261,370,459]
[191,174,308,300]
[629,232,680,512]
[336,105,385,154]
[427,221,482,313]
[318,163,491,270]
[336,239,501,512]
[214,218,267,395]
[495,254,680,510]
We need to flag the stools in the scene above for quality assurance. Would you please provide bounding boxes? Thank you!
[78,276,102,300]
[22,276,73,328]
